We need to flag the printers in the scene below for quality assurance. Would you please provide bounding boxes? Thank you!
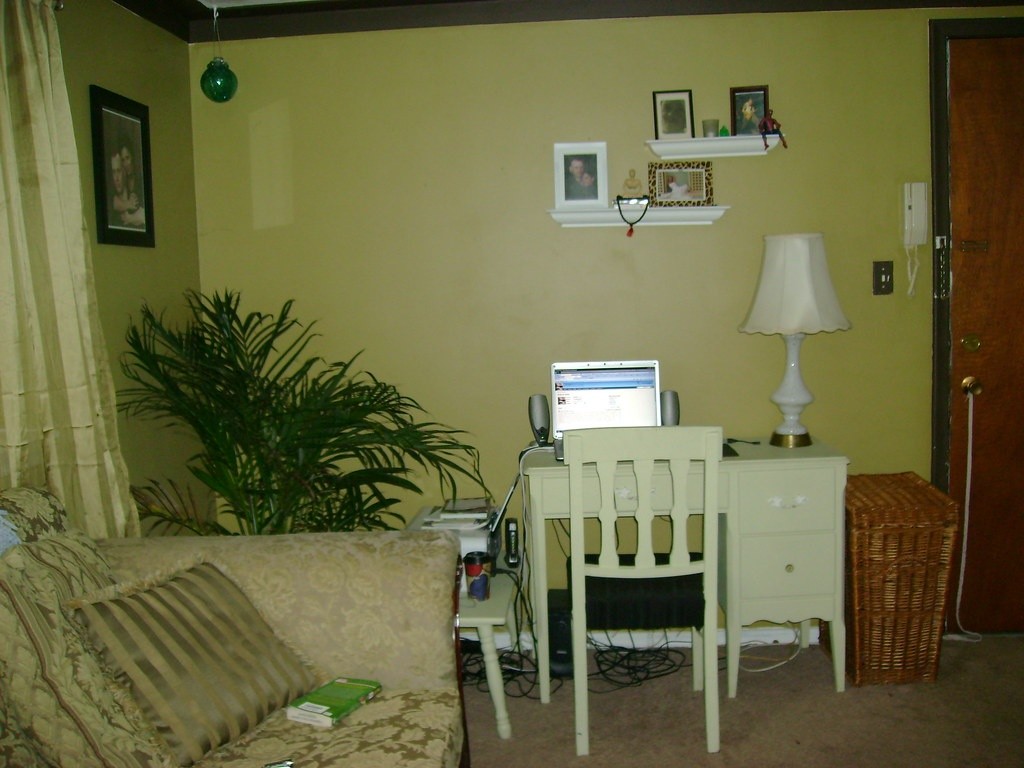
[404,475,520,561]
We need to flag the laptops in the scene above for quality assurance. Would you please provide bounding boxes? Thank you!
[551,359,662,461]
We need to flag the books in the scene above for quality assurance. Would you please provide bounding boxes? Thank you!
[286,676,382,728]
[420,496,491,532]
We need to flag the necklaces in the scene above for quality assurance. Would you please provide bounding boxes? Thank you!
[617,195,650,236]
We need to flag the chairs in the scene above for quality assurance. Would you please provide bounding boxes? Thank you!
[564,427,723,754]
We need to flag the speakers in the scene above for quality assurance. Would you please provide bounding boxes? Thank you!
[528,394,550,446]
[660,390,680,425]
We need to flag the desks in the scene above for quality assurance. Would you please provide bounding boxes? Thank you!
[409,511,523,737]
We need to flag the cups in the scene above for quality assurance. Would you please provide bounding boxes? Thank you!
[461,552,494,601]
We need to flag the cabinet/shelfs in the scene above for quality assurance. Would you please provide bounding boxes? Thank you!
[519,448,851,698]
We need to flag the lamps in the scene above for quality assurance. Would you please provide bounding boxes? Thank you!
[738,233,851,448]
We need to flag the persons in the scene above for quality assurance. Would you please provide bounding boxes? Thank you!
[661,100,687,134]
[566,157,597,199]
[741,99,755,134]
[103,137,146,234]
[758,109,788,150]
[667,176,687,193]
[622,169,643,194]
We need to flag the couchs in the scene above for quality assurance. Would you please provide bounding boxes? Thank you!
[0,484,469,768]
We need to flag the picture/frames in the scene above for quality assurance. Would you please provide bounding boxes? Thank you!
[730,85,770,136]
[647,161,714,207]
[653,89,695,140]
[554,142,608,211]
[89,84,155,248]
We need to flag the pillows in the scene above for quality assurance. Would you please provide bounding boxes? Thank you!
[66,557,320,768]
